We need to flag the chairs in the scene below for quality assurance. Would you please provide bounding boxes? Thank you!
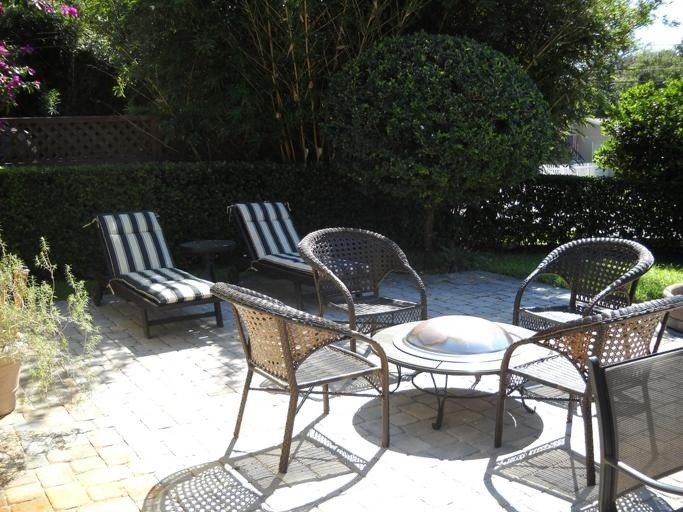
[208,281,395,477]
[509,234,652,414]
[80,210,224,337]
[487,290,681,486]
[295,221,431,384]
[582,343,682,512]
[225,202,373,310]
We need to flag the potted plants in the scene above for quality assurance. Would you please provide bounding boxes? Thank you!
[0,225,105,419]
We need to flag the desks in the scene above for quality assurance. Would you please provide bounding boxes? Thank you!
[179,239,239,289]
[353,315,553,432]
